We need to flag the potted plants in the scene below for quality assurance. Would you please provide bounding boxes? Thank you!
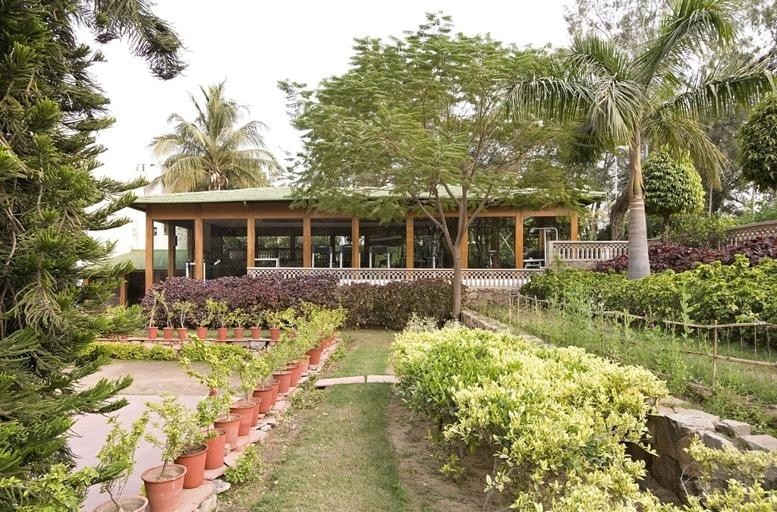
[192,395,226,469]
[138,391,194,511]
[172,418,207,490]
[169,298,198,339]
[203,300,240,342]
[265,311,282,339]
[228,311,249,340]
[154,291,175,339]
[228,296,349,436]
[89,410,152,512]
[179,351,241,451]
[245,309,264,339]
[141,293,160,338]
[186,309,211,340]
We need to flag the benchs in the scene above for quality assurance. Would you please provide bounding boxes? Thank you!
[523,259,545,268]
